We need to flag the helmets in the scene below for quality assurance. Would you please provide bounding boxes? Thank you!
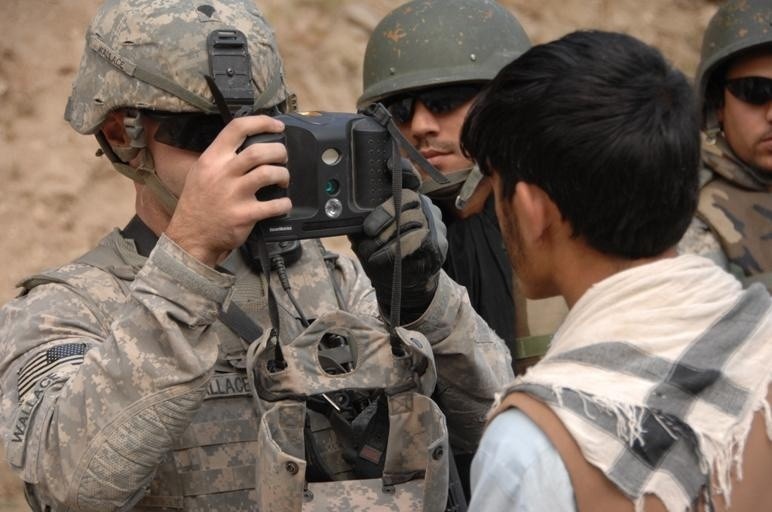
[63,1,293,135]
[695,2,772,117]
[353,1,536,112]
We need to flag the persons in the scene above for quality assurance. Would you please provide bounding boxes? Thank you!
[350,2,567,376]
[675,0,771,302]
[442,27,771,510]
[0,1,516,511]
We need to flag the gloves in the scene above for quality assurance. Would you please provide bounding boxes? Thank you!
[344,188,450,329]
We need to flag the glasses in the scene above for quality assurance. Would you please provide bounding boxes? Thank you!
[136,102,241,158]
[717,76,772,109]
[379,80,492,128]
